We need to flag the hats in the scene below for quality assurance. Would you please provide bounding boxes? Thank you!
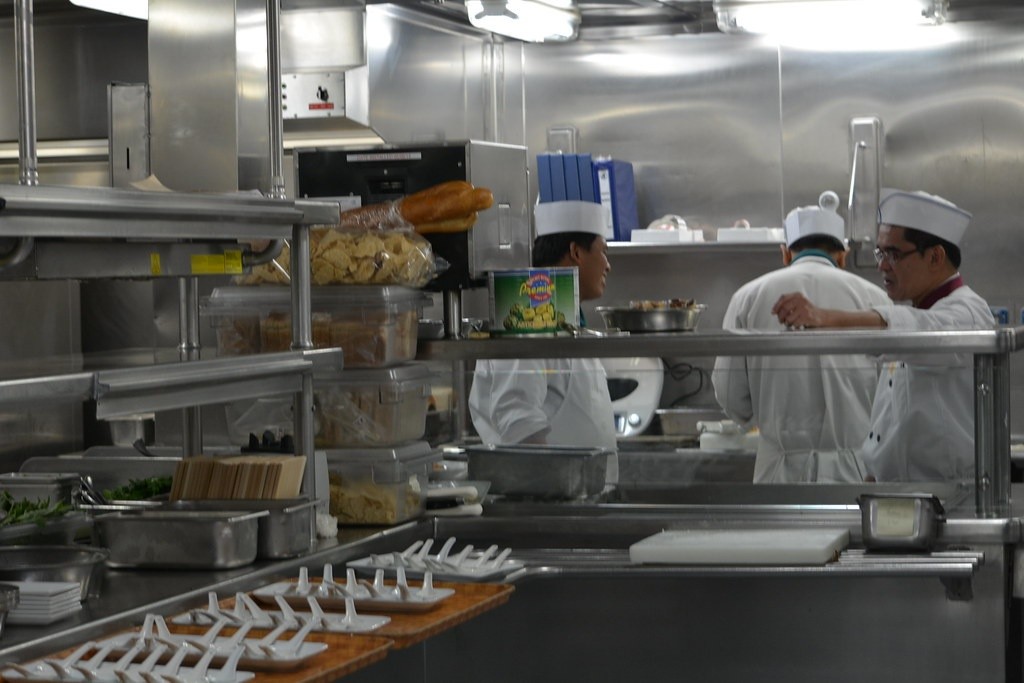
[783,205,847,252]
[533,200,609,239]
[877,186,972,245]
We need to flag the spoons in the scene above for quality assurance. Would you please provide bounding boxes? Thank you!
[6,537,512,683]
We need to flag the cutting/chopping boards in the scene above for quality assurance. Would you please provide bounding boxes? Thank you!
[615,435,701,452]
[630,529,850,568]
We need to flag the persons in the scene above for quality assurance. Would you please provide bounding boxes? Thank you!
[711,206,894,483]
[469,201,619,501]
[771,188,996,518]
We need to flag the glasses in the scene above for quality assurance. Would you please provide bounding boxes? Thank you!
[871,245,930,263]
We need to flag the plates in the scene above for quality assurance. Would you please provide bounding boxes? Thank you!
[595,305,703,334]
[0,551,525,683]
[1,579,83,625]
